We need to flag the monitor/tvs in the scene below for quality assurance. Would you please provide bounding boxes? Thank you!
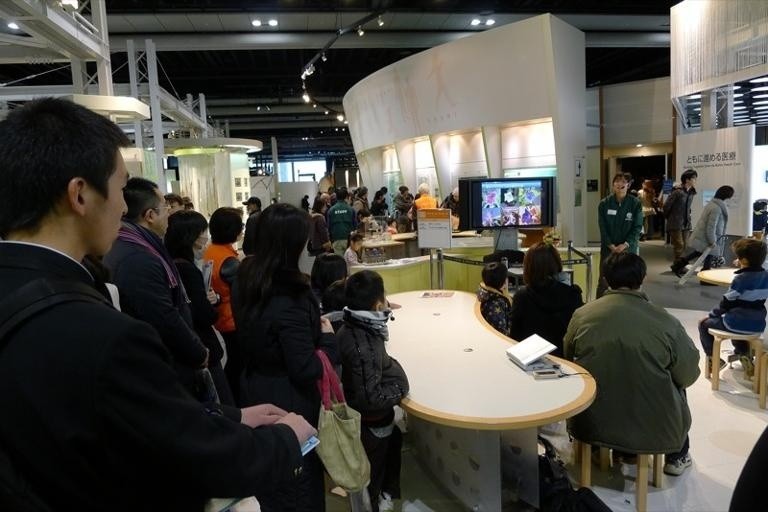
[468,176,557,264]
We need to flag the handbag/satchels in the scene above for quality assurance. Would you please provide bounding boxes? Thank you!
[538,455,613,512]
[315,400,371,493]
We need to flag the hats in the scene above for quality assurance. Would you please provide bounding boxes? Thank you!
[242,197,261,205]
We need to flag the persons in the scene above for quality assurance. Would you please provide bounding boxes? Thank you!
[392,183,458,232]
[762,202,768,235]
[480,261,514,337]
[562,253,699,474]
[597,171,643,299]
[671,184,735,279]
[752,200,767,232]
[699,237,768,380]
[0,97,410,511]
[628,171,673,241]
[512,241,584,356]
[663,170,699,275]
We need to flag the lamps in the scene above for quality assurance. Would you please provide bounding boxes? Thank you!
[251,18,497,125]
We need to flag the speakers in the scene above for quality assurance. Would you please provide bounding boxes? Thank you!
[163,155,179,169]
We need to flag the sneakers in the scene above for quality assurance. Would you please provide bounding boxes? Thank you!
[664,454,692,475]
[740,356,754,376]
[709,359,727,373]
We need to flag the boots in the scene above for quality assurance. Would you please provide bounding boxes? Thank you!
[671,257,689,278]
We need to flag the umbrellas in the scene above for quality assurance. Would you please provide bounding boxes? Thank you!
[677,234,727,290]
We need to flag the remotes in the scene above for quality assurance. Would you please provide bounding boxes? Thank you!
[532,369,562,379]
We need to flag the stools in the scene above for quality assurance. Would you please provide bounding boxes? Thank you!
[574,327,768,512]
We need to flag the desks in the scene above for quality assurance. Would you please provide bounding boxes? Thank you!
[385,288,597,511]
[697,267,768,368]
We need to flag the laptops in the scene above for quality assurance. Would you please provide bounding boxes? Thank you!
[505,333,563,371]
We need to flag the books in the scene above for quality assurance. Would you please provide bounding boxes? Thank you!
[417,291,456,298]
